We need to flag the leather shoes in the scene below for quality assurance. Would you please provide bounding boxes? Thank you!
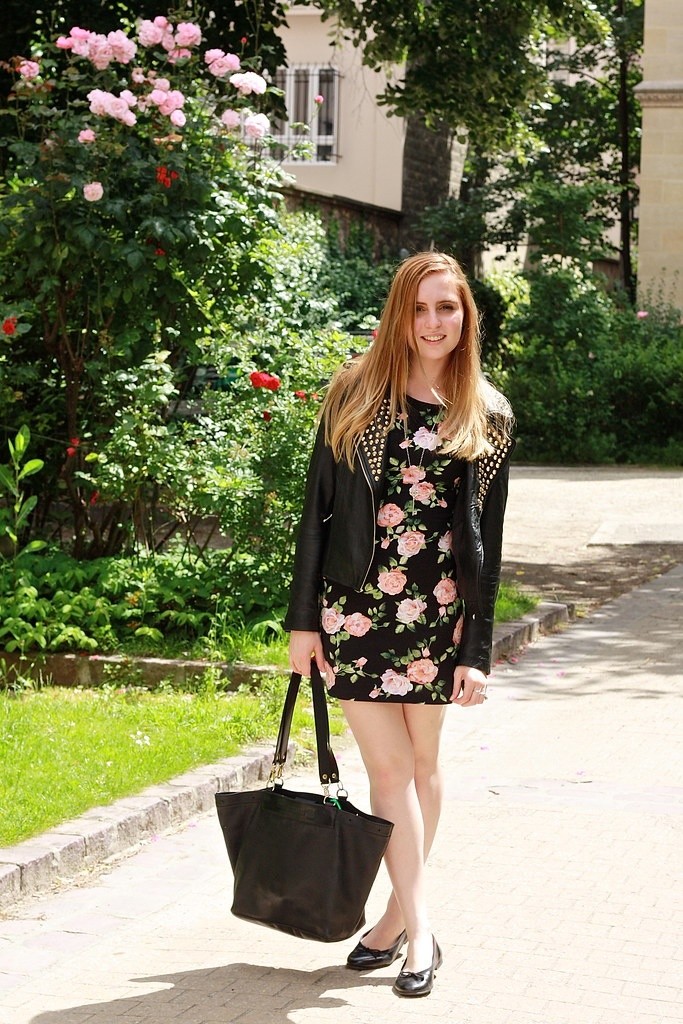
[396,935,444,995]
[347,931,408,968]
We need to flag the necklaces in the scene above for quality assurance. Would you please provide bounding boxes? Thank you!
[405,403,445,513]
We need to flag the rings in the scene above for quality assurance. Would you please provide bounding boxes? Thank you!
[474,689,488,700]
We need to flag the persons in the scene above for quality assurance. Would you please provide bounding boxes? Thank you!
[280,251,516,997]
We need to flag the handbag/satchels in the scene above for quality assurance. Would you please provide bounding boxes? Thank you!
[214,664,393,945]
